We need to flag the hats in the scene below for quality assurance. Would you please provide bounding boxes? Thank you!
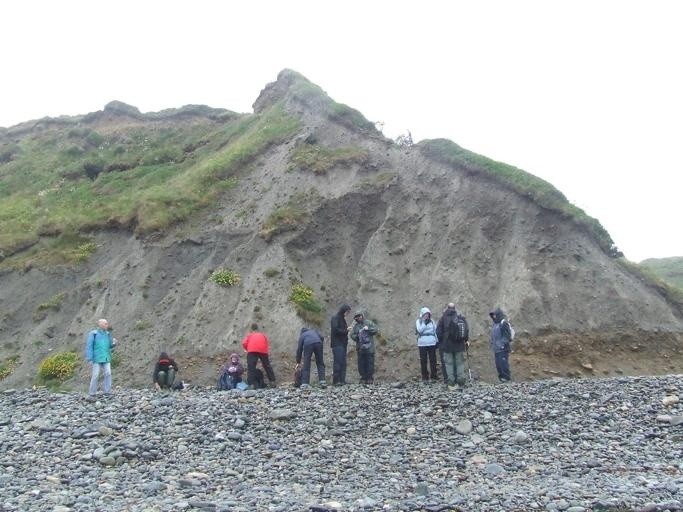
[353,311,365,320]
[230,353,240,363]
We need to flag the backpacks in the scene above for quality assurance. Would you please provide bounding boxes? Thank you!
[501,319,515,340]
[358,329,371,349]
[449,316,466,343]
[294,363,304,386]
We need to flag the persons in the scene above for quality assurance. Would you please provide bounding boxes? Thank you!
[86,318,119,395]
[153,352,178,390]
[489,308,514,383]
[415,307,439,382]
[331,303,351,386]
[436,302,469,391]
[219,353,244,390]
[350,311,377,384]
[243,323,276,390]
[295,328,326,387]
